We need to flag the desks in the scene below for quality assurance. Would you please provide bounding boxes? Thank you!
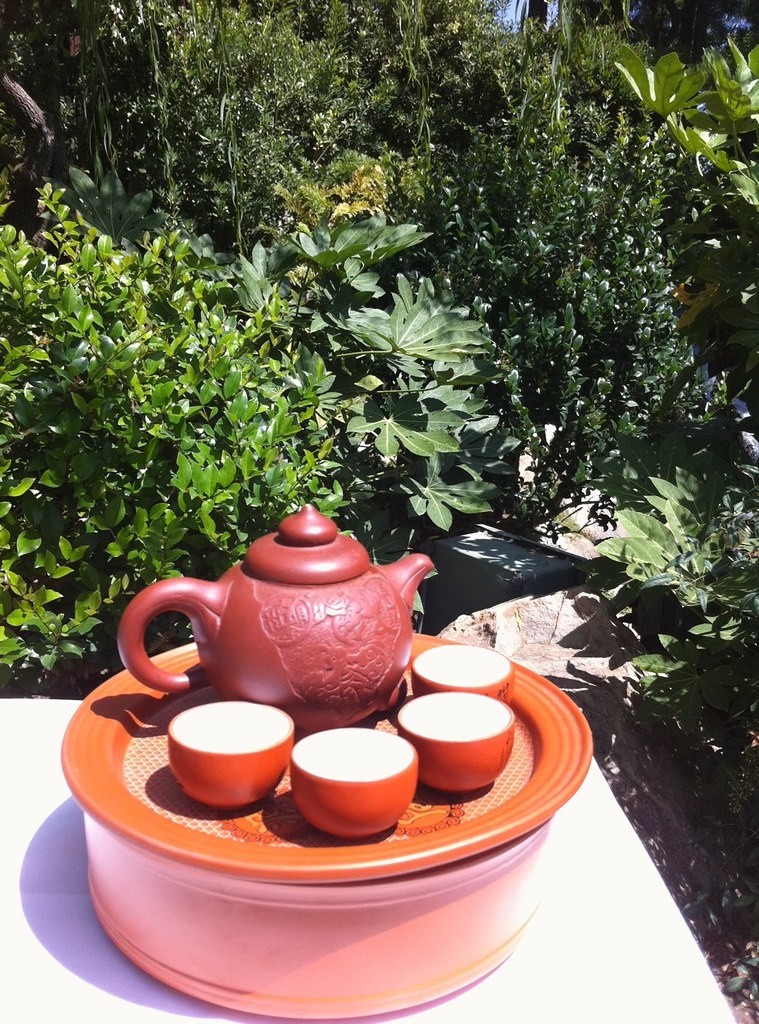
[0,699,736,1024]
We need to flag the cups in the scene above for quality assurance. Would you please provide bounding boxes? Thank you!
[167,701,294,810]
[291,727,417,839]
[398,693,515,794]
[415,645,512,707]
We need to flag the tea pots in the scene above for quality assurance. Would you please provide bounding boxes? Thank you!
[116,506,436,732]
